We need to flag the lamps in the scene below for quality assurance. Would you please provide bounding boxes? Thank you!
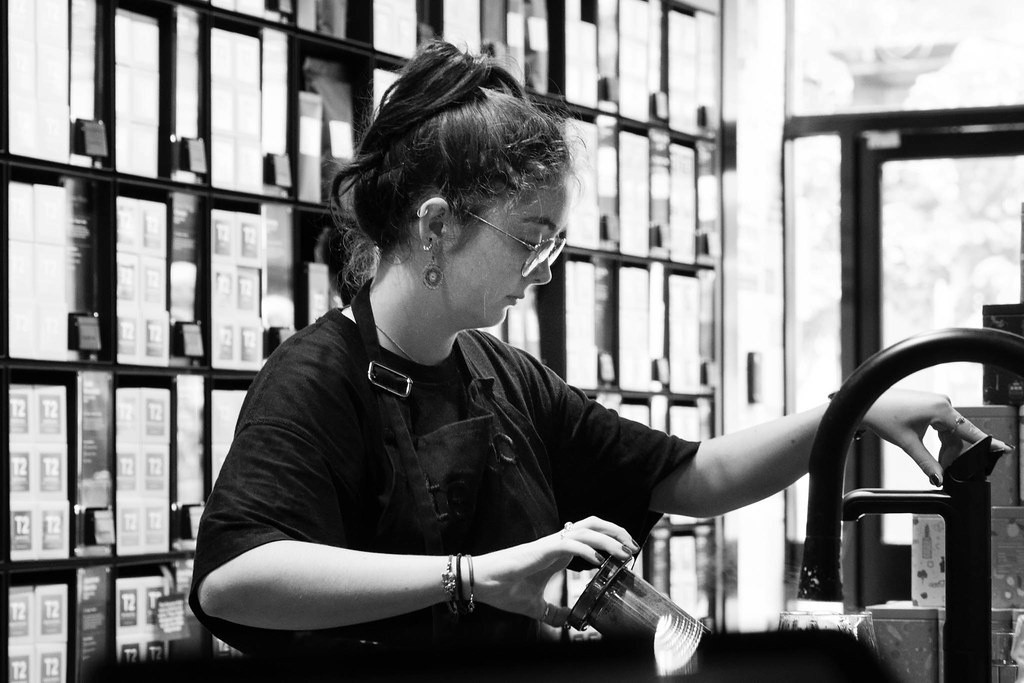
[567,555,705,677]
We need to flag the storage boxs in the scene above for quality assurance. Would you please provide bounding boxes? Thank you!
[864,405,1024,683]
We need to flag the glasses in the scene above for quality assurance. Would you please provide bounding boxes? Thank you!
[460,209,566,278]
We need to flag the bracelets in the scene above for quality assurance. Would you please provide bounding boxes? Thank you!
[440,553,475,619]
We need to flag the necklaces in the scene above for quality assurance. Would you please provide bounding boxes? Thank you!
[376,324,420,364]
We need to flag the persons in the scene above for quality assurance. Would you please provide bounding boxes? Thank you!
[185,34,1018,683]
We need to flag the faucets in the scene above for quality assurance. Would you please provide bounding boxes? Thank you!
[840,433,1006,683]
